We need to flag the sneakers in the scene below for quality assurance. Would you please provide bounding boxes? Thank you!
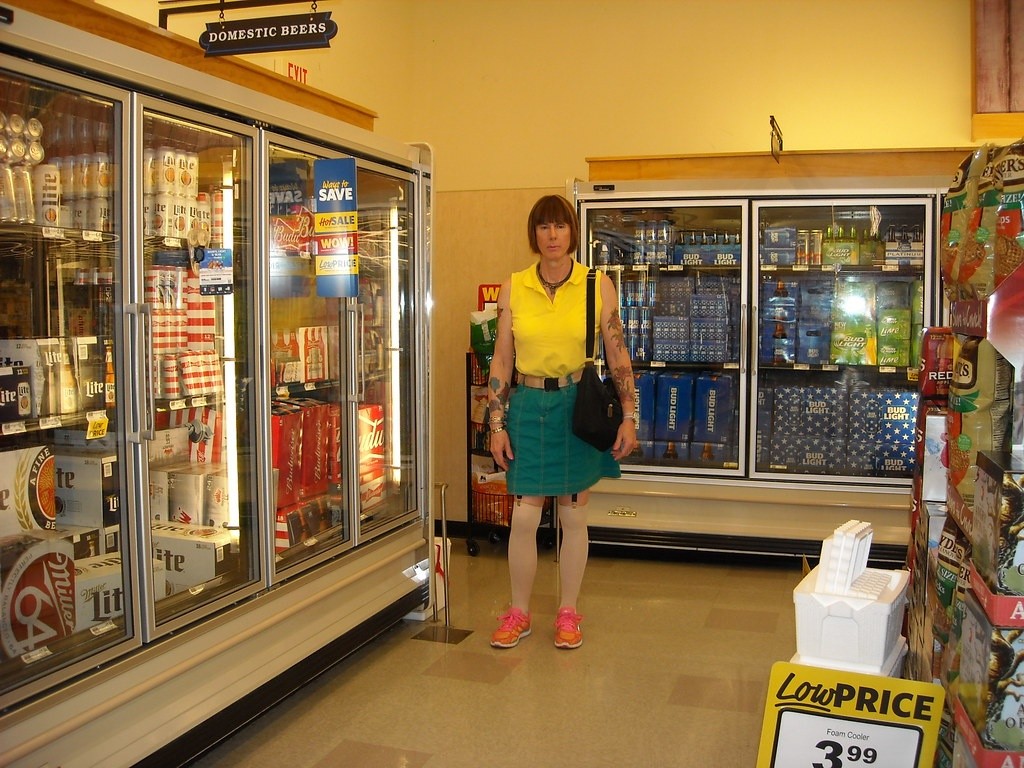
[552,606,583,648]
[491,606,531,647]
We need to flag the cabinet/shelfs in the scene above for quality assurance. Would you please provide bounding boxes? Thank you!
[463,351,554,558]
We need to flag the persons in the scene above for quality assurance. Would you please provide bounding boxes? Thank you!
[490,195,639,650]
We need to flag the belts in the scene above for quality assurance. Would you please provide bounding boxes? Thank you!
[516,370,584,391]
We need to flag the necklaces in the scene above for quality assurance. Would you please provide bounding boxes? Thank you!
[538,258,573,295]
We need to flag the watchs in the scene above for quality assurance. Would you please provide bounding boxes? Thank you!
[624,412,639,424]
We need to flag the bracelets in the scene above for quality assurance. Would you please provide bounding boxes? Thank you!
[490,426,506,433]
[489,417,506,424]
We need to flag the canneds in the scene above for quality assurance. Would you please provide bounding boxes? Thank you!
[0,111,225,399]
[601,220,923,367]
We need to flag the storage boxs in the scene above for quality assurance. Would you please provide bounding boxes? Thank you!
[601,228,1024,768]
[0,323,392,662]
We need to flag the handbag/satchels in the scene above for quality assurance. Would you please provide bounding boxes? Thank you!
[571,366,623,452]
